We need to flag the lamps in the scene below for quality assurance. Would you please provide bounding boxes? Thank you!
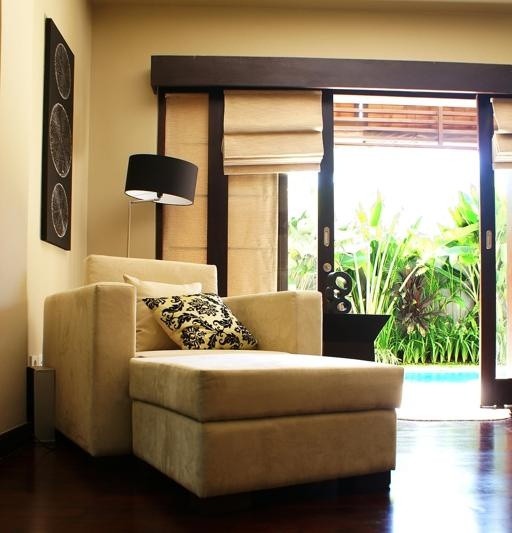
[123,153,198,258]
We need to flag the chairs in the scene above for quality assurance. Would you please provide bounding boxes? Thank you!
[43,255,322,456]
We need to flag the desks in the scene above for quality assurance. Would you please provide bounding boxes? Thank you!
[323,313,391,362]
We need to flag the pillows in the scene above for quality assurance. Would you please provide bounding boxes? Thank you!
[122,273,203,351]
[141,292,260,350]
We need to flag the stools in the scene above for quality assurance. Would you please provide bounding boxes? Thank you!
[128,352,404,515]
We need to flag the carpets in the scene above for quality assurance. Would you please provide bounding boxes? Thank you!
[396,408,510,421]
